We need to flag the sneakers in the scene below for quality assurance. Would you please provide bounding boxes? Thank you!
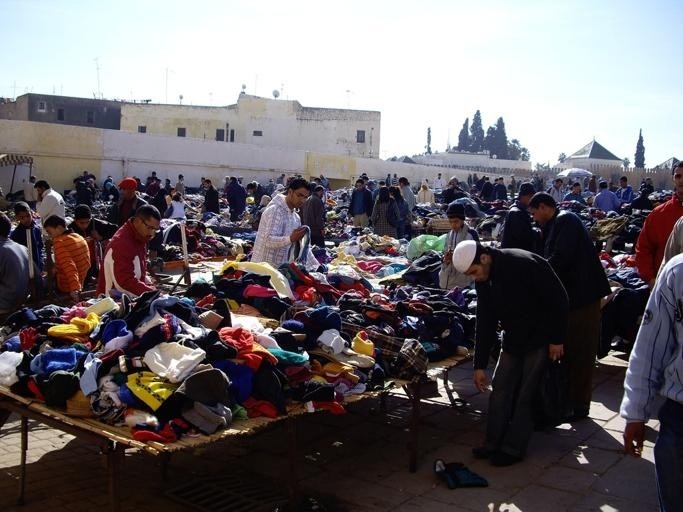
[470,441,526,467]
[559,403,589,419]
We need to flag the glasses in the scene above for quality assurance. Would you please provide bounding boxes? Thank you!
[139,217,162,233]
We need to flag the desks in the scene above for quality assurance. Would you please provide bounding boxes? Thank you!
[1,329,475,512]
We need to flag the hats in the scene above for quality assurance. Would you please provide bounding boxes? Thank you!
[118,177,138,190]
[451,240,477,274]
[445,203,466,220]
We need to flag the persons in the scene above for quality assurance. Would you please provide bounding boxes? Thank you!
[451,237,571,469]
[634,159,683,291]
[524,189,614,429]
[617,250,682,512]
[653,217,683,291]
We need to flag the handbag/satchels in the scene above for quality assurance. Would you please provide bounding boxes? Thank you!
[387,198,399,229]
